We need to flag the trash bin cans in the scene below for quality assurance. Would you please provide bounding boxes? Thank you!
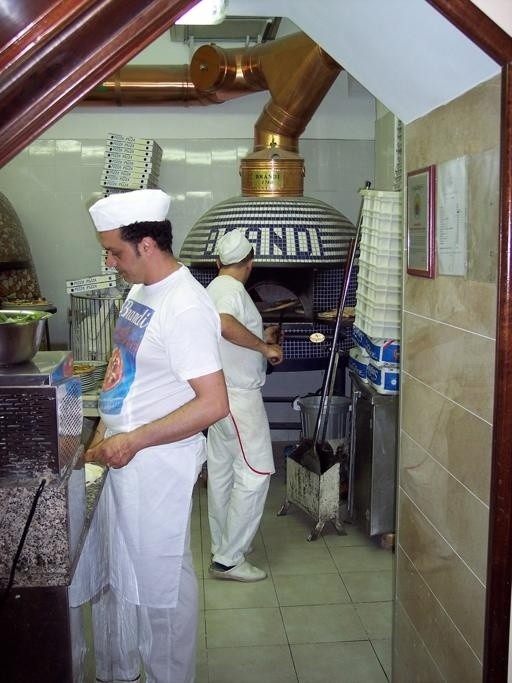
[297,396,350,442]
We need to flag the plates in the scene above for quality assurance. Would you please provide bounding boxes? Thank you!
[71,360,107,393]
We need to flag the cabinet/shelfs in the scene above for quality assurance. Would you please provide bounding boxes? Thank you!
[348,371,396,537]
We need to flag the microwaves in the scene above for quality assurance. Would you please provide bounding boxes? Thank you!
[0,352,84,487]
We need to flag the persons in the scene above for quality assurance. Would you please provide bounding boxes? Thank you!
[199,228,286,584]
[52,349,112,683]
[81,187,231,683]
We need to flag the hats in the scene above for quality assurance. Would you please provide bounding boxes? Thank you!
[88,188,171,232]
[217,228,253,266]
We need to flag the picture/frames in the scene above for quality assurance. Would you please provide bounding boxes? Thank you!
[405,165,436,278]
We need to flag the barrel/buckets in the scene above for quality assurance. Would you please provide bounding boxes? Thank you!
[297,396,353,493]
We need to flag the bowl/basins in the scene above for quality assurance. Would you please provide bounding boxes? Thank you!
[0,307,53,366]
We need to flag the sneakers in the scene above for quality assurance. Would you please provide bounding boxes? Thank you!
[209,546,267,584]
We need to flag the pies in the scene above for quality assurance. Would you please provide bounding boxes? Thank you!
[318,307,355,318]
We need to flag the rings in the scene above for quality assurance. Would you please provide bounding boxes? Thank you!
[106,461,111,468]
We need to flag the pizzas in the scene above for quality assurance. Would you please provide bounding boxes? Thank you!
[74,365,96,374]
